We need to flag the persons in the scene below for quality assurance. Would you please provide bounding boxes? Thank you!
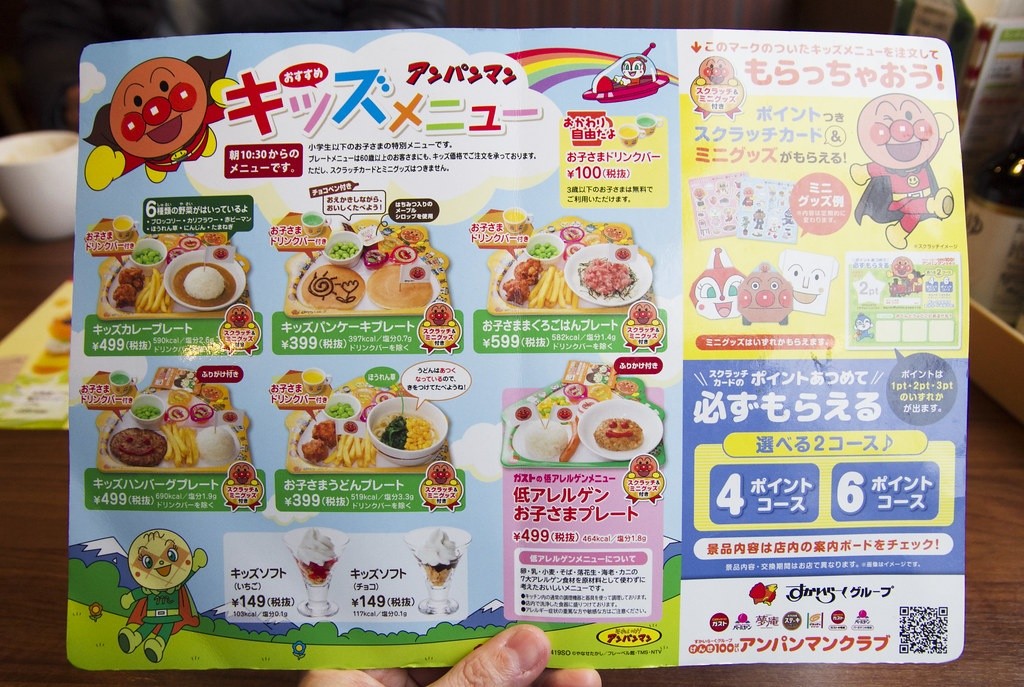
[293,622,604,687]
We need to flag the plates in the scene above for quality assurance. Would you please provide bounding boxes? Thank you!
[98,363,665,484]
[564,245,653,306]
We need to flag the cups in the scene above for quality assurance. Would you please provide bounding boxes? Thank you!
[405,527,471,616]
[302,213,324,236]
[286,528,341,618]
[503,208,533,232]
[0,132,82,240]
[111,215,140,242]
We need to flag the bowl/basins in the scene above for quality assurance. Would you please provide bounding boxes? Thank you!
[324,232,363,269]
[364,396,449,467]
[128,240,167,270]
[525,233,566,264]
[163,248,246,310]
